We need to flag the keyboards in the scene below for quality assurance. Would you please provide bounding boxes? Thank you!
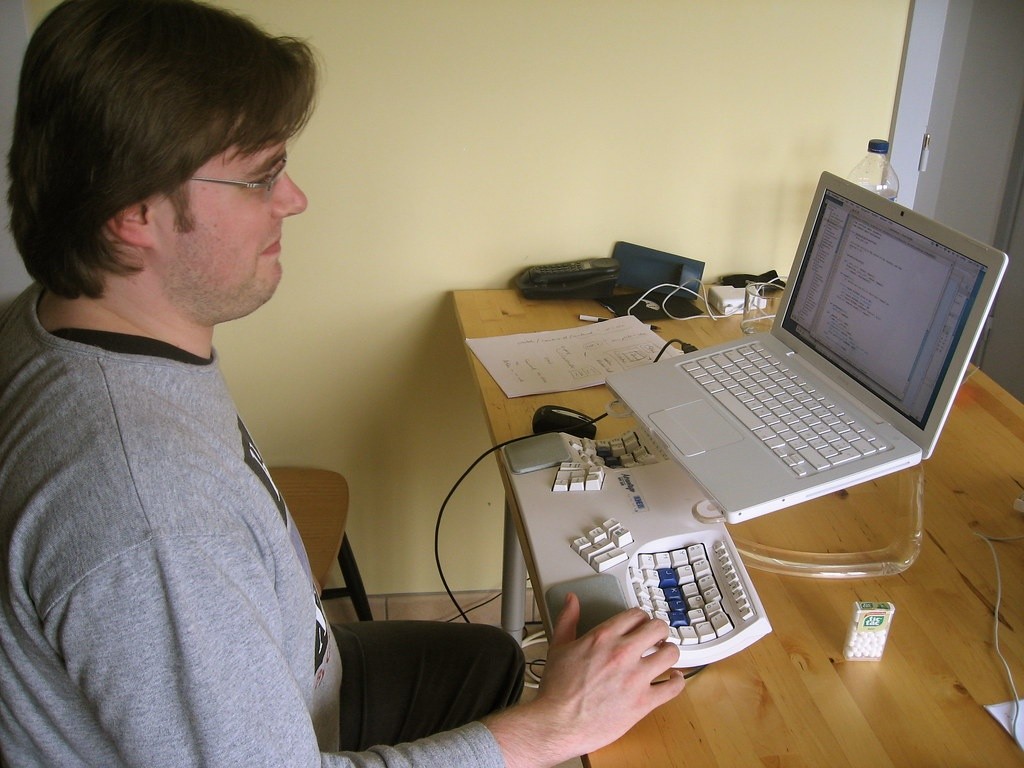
[500,429,772,670]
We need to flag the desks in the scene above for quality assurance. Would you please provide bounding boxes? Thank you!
[451,277,1024,767]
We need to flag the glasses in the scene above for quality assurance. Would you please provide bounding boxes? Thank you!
[188,154,287,191]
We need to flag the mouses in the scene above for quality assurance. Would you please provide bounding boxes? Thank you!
[531,405,597,440]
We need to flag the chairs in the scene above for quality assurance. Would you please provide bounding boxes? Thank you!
[267,466,375,621]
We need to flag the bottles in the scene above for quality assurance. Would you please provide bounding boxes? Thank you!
[848,139,900,205]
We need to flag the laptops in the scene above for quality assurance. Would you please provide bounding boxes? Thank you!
[605,171,1010,527]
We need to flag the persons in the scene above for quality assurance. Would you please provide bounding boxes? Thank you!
[0,1,685,768]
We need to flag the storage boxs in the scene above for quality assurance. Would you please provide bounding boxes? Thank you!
[843,602,895,661]
